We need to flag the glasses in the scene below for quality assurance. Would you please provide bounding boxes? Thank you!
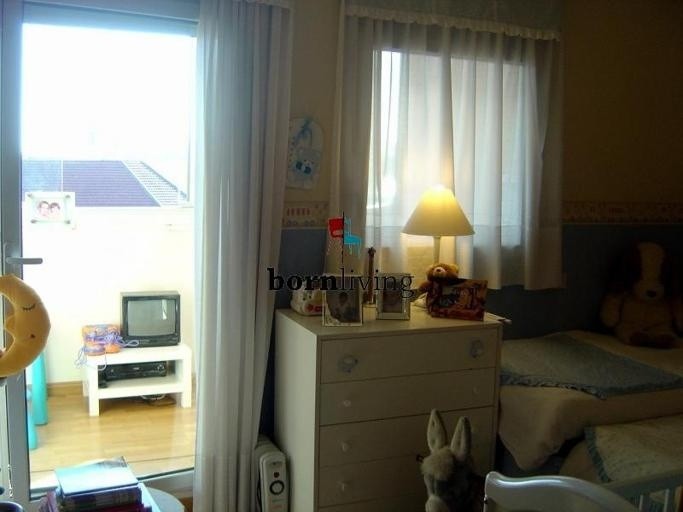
[403,187,475,306]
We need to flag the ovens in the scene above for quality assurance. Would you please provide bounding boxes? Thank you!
[493,330,683,512]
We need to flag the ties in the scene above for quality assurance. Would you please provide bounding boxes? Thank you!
[122,290,181,347]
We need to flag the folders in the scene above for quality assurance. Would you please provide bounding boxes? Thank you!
[252,434,289,512]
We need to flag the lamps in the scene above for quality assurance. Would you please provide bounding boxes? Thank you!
[482,470,639,512]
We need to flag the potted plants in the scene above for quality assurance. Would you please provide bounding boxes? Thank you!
[430,280,487,320]
[375,273,410,321]
[321,273,364,327]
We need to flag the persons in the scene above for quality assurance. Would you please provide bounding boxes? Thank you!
[333,292,353,322]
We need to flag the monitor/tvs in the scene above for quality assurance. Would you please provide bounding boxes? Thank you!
[53,452,153,512]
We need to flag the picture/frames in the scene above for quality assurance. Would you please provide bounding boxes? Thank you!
[83,345,192,418]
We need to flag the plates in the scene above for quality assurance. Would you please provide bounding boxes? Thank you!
[273,307,503,512]
